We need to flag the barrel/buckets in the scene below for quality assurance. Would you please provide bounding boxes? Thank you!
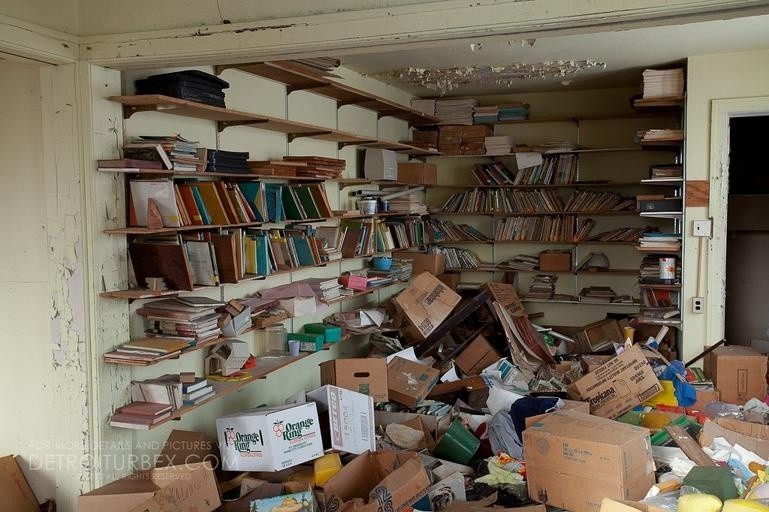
[434,420,480,461]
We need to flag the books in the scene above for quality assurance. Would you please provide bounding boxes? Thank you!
[99,67,687,428]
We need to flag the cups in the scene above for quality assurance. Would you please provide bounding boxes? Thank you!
[287,338,300,358]
[658,257,676,281]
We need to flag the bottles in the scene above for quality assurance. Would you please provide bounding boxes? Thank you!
[253,323,289,360]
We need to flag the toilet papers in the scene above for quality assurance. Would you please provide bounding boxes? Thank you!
[660,257,675,279]
